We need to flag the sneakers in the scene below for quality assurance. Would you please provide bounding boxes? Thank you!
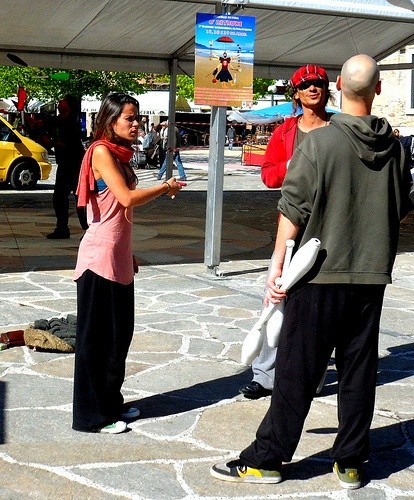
[329,449,361,490]
[121,406,140,419]
[209,458,281,484]
[99,420,128,434]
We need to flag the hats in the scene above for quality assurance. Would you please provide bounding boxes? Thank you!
[160,121,167,125]
[289,63,329,91]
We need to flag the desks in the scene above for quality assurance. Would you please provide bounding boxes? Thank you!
[241,143,268,167]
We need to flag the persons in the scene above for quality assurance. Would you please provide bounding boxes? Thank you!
[134,116,186,181]
[240,65,333,398]
[72,91,181,434]
[32,94,87,240]
[227,125,236,150]
[394,129,403,141]
[210,54,414,488]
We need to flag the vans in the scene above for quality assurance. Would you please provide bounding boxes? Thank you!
[0,115,53,191]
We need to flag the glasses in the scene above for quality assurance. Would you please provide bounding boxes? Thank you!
[298,79,325,90]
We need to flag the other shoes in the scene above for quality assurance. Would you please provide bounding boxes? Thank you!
[157,176,161,180]
[181,177,186,181]
[150,165,157,169]
[46,227,71,239]
[238,380,269,399]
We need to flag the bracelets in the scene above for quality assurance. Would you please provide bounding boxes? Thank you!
[162,182,170,195]
